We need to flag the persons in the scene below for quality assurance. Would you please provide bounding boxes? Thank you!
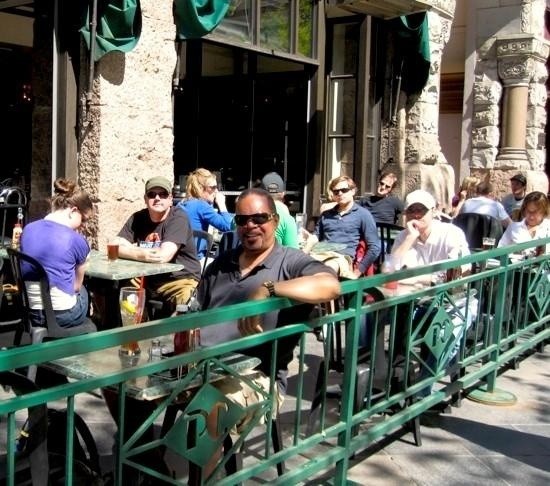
[448,173,550,352]
[361,170,404,271]
[15,177,109,331]
[312,178,381,279]
[177,167,235,273]
[145,189,339,486]
[113,176,202,319]
[262,172,318,257]
[354,190,477,408]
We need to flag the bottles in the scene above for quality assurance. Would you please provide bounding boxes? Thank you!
[149,339,162,363]
[12,224,22,243]
[174,305,189,354]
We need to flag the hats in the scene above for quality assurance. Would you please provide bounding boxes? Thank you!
[510,174,527,184]
[403,190,435,212]
[145,175,172,194]
[262,171,284,193]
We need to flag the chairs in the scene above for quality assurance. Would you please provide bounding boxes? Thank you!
[0,214,550,486]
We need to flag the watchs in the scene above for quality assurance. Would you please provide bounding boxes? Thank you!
[263,279,276,295]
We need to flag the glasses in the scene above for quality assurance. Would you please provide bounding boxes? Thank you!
[146,191,168,199]
[205,184,218,191]
[333,188,352,195]
[235,213,273,226]
[78,209,88,223]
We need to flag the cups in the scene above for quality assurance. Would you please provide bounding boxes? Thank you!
[107,238,119,262]
[118,287,146,355]
[384,254,399,289]
[482,237,495,251]
[296,212,308,245]
